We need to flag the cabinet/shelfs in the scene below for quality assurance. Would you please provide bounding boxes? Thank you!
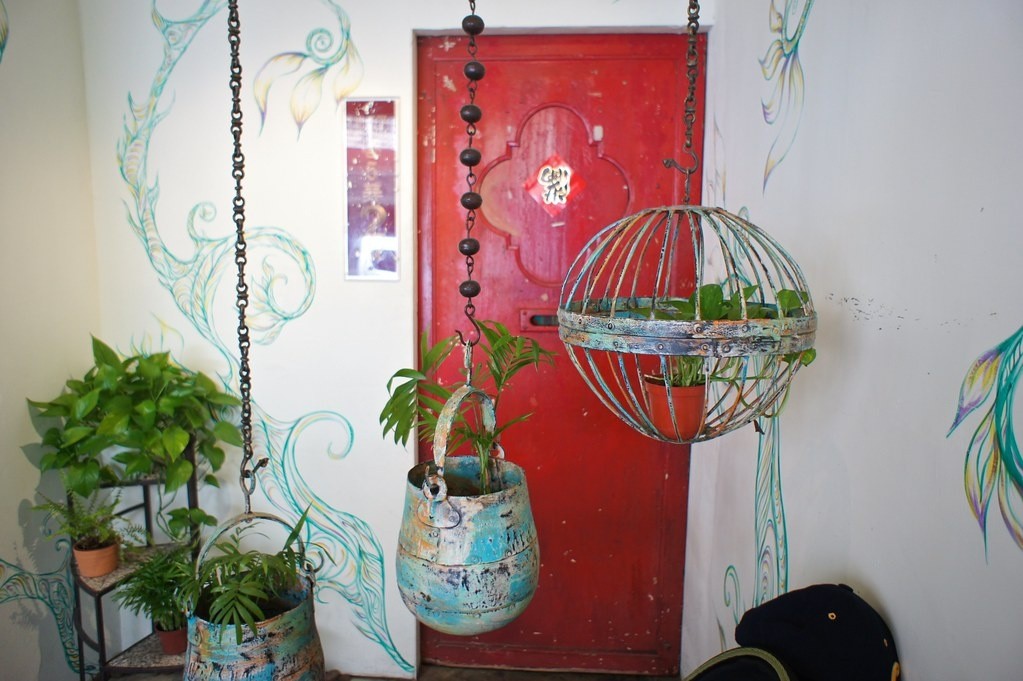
[66,432,201,681]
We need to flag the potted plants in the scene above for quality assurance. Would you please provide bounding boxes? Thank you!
[110,501,337,680]
[632,284,817,441]
[379,320,558,636]
[27,468,156,579]
[25,332,245,551]
